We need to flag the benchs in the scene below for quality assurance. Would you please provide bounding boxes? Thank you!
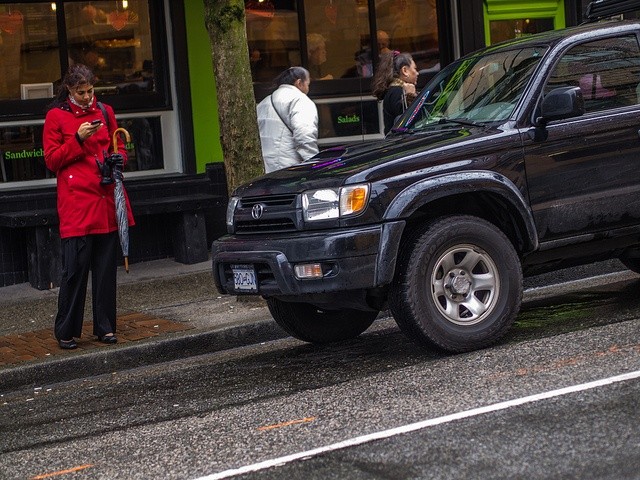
[0,193,229,289]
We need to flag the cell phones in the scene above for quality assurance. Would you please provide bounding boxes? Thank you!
[91,120,101,125]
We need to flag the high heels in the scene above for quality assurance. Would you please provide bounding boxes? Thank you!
[99,333,117,343]
[58,336,76,349]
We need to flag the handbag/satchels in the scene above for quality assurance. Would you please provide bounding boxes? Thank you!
[394,107,430,129]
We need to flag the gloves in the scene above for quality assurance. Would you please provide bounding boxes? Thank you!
[108,153,124,169]
[96,149,112,185]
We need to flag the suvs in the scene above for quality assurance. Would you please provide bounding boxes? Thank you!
[211,20,640,355]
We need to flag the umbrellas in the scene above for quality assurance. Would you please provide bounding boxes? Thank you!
[112,127,132,273]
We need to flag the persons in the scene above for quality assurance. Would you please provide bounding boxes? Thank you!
[372,51,423,136]
[42,63,136,350]
[306,33,334,80]
[257,66,319,175]
[250,50,260,64]
[377,30,390,54]
